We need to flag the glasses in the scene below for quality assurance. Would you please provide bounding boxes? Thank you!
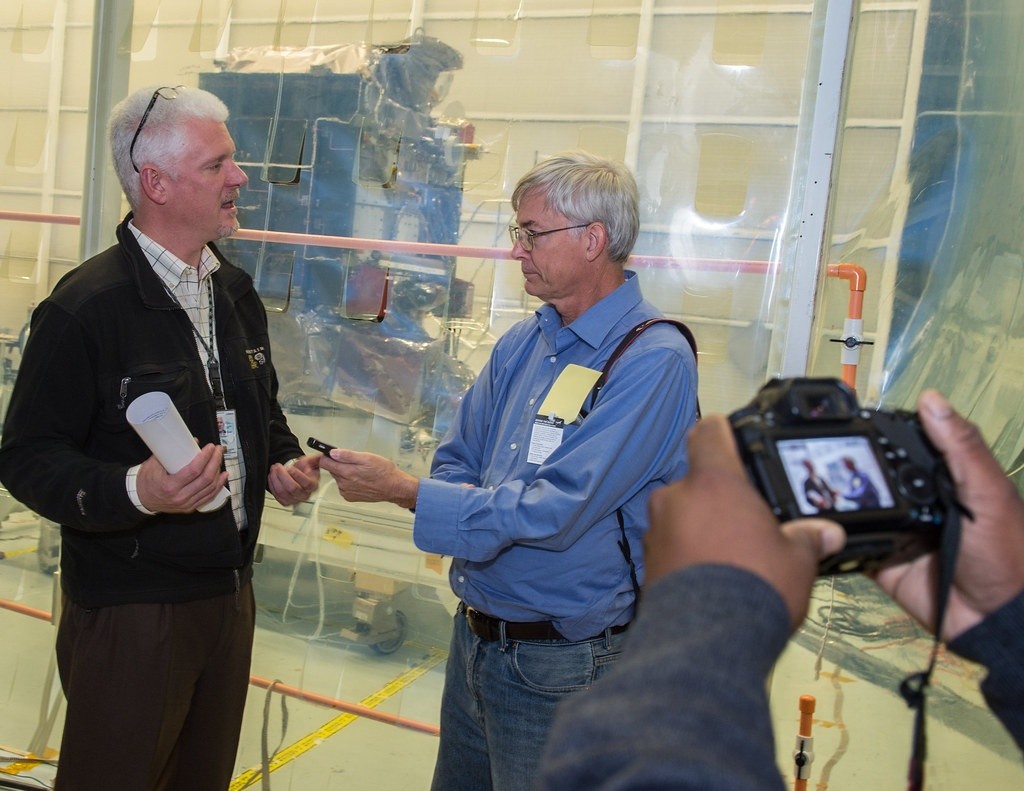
[508,221,593,252]
[130,84,187,174]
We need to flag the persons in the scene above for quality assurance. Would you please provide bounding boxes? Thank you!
[0,85,321,791]
[537,387,1024,791]
[802,458,839,514]
[319,152,697,791]
[840,456,882,510]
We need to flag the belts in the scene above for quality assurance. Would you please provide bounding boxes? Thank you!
[457,600,631,642]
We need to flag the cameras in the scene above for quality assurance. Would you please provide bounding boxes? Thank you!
[725,376,962,576]
